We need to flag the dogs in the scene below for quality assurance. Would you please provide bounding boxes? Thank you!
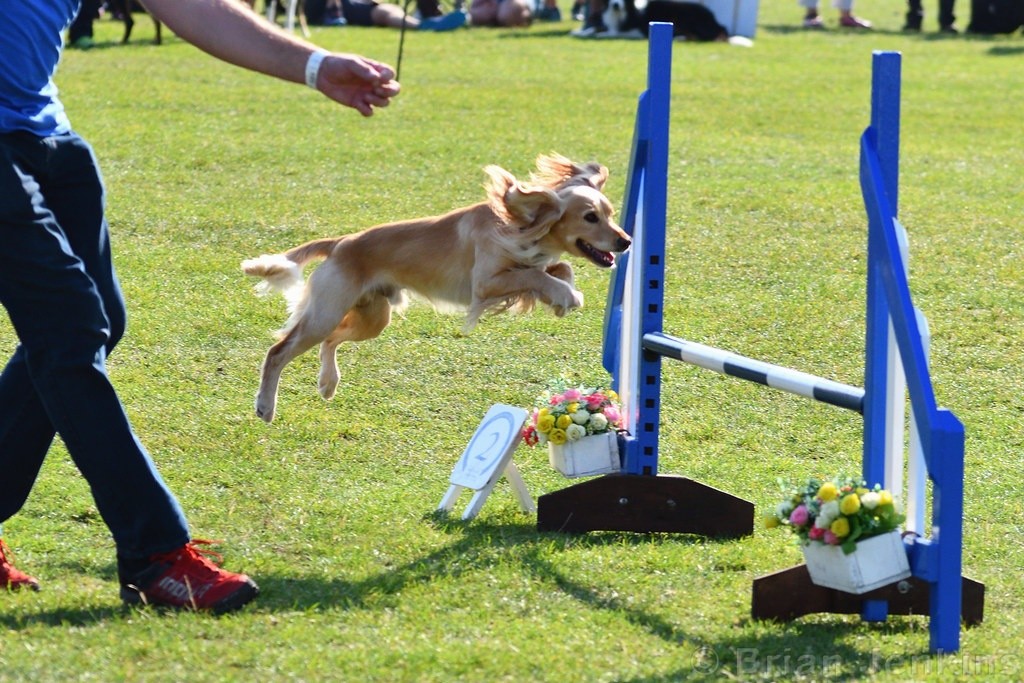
[240,150,632,422]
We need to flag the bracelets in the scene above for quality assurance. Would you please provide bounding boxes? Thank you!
[305,48,331,90]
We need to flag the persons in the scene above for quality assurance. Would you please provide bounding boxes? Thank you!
[242,0,642,37]
[0,0,400,618]
[903,0,958,33]
[799,0,873,29]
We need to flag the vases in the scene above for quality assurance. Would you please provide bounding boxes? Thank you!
[547,431,621,479]
[801,528,911,594]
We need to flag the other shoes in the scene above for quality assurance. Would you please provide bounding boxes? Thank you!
[325,6,347,25]
[840,17,872,28]
[803,15,823,28]
[418,11,465,32]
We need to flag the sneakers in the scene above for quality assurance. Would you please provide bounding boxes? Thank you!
[0,539,42,592]
[73,35,95,49]
[120,539,260,617]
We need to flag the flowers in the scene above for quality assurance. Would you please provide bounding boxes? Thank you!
[759,469,906,557]
[521,383,625,447]
